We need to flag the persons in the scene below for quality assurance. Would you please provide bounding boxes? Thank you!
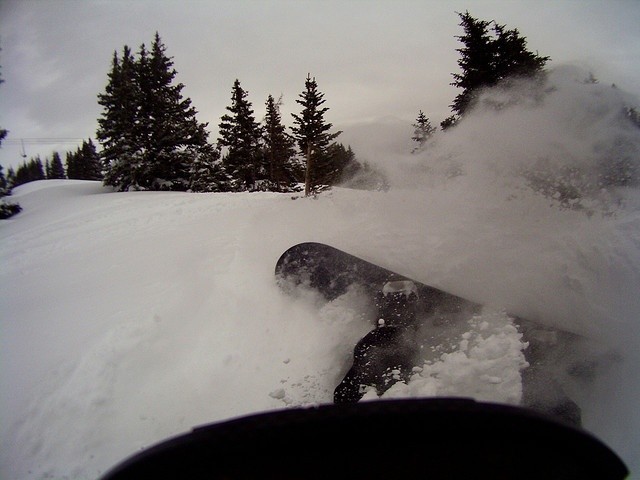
[98,280,631,480]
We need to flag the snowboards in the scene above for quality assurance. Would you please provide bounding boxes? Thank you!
[274,241,602,415]
